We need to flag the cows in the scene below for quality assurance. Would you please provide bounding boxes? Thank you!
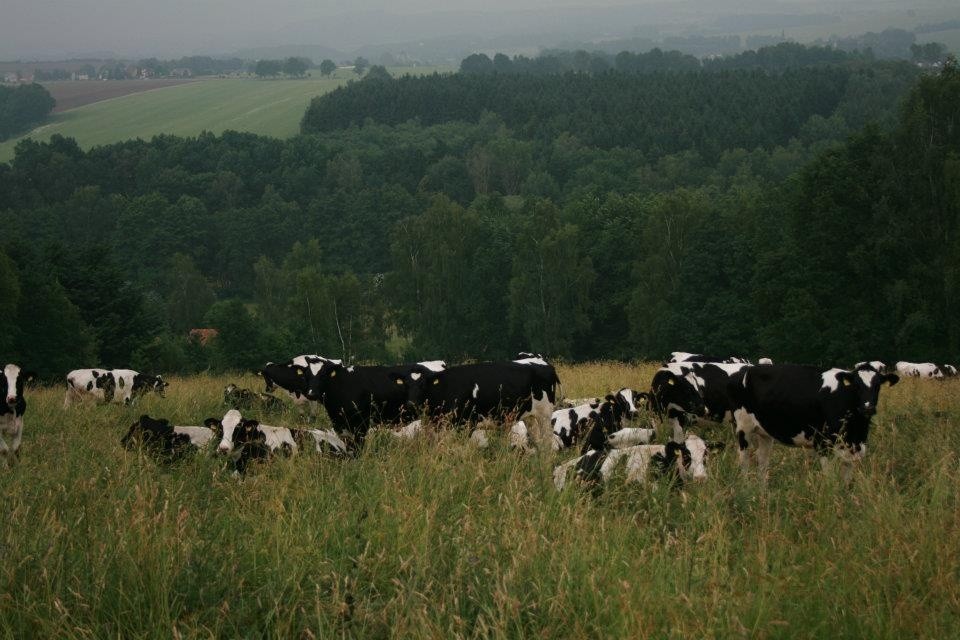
[0,364,32,470]
[259,354,658,450]
[120,410,349,482]
[61,368,170,410]
[223,383,289,415]
[896,362,959,381]
[552,435,704,498]
[647,351,900,486]
